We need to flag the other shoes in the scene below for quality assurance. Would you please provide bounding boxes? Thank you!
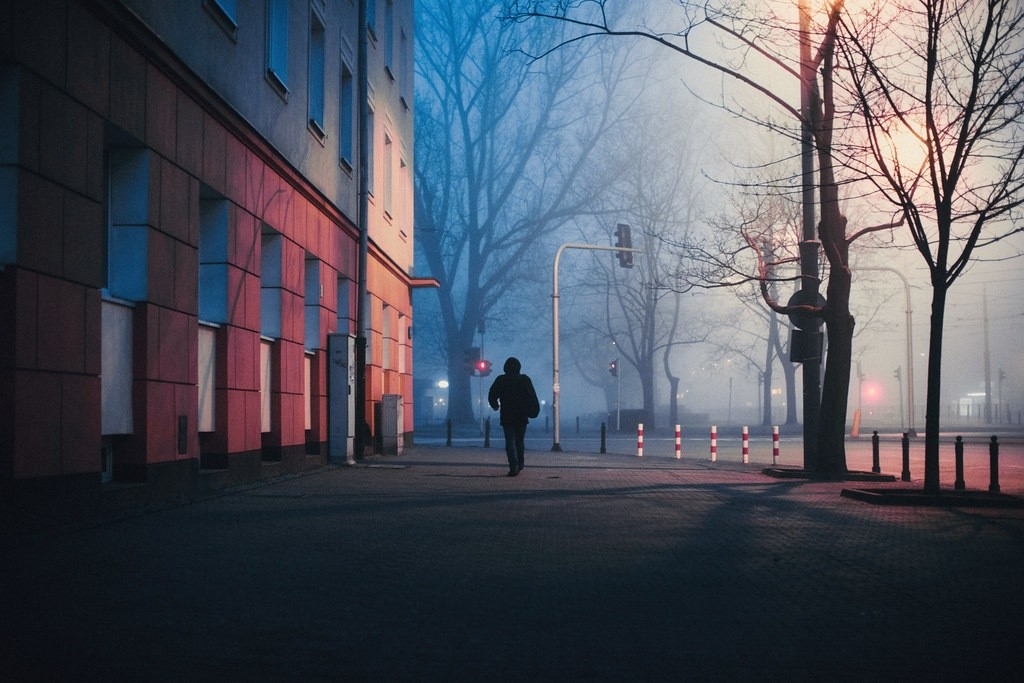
[508,462,525,477]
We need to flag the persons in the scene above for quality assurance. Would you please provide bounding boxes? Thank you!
[488,356,536,477]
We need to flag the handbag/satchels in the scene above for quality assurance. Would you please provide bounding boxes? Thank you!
[522,374,541,418]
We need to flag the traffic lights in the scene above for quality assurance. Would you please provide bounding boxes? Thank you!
[758,373,764,385]
[859,372,864,383]
[614,222,634,269]
[609,360,616,375]
[894,368,900,380]
[462,347,480,378]
[479,361,493,376]
[999,369,1006,381]
